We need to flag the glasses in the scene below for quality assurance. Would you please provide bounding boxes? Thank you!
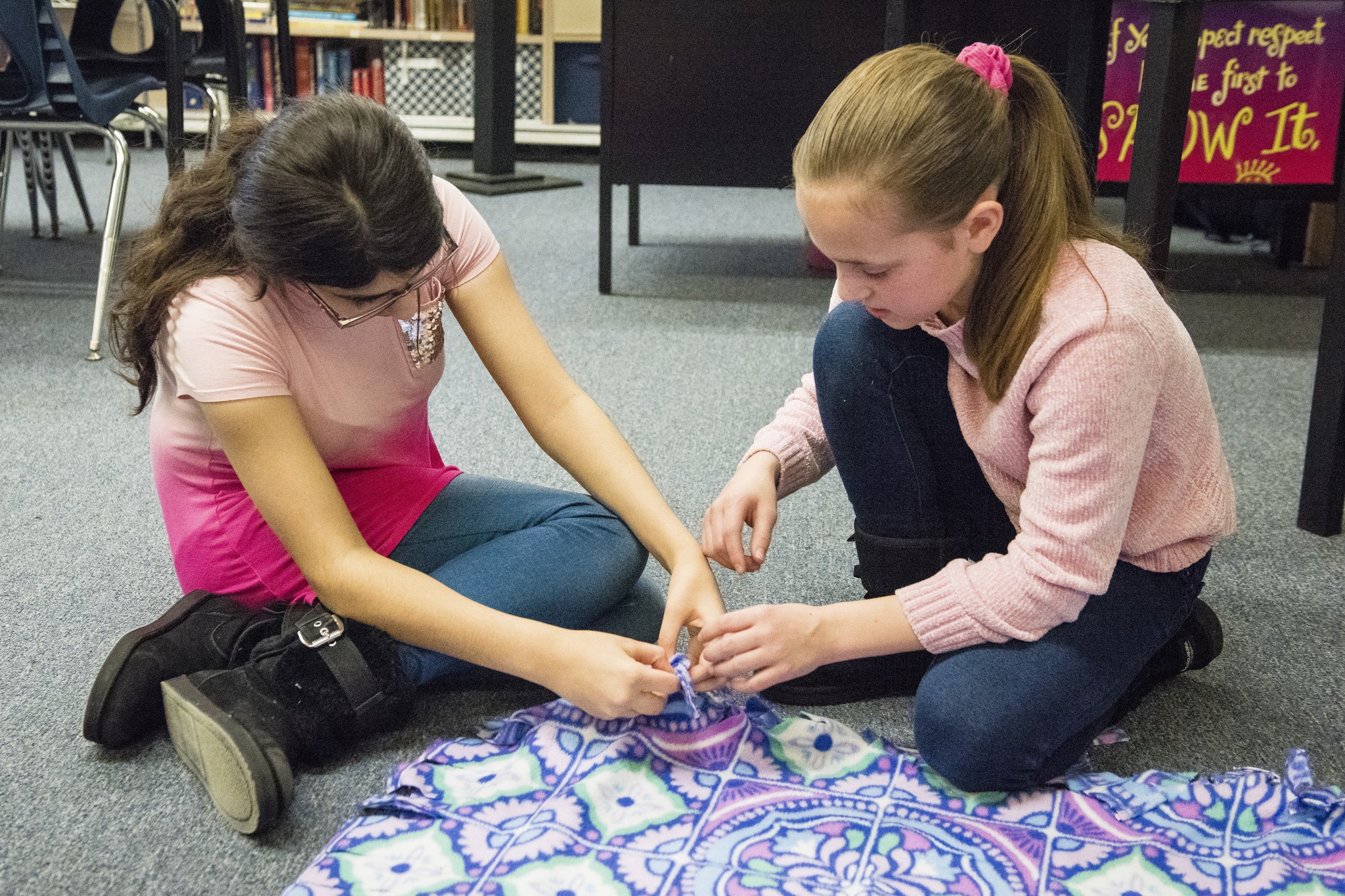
[302,227,459,329]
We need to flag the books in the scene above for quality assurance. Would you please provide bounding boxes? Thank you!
[368,0,473,31]
[182,32,384,112]
[243,0,368,20]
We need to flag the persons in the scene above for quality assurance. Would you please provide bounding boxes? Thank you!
[697,28,1237,792]
[84,89,751,837]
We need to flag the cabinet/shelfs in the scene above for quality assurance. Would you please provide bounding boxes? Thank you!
[48,0,602,152]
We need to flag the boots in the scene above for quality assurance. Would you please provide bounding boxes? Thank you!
[161,607,411,837]
[760,516,1015,705]
[1112,601,1222,721]
[82,591,287,755]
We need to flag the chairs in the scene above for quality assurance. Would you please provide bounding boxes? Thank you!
[0,0,232,364]
